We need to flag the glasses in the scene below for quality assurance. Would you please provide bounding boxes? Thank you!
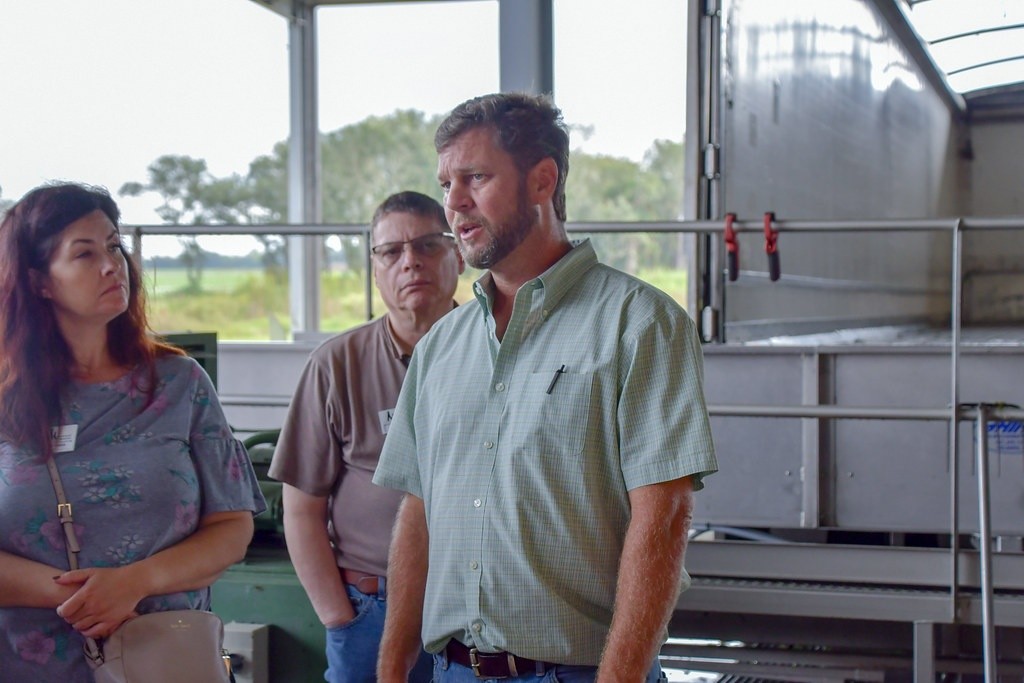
[370,232,455,264]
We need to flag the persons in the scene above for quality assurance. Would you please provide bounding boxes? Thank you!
[268,188,466,683]
[371,93,720,683]
[0,185,267,683]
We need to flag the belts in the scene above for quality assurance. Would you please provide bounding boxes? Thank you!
[438,637,559,680]
[341,569,379,595]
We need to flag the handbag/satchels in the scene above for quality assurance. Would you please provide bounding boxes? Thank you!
[82,609,238,683]
